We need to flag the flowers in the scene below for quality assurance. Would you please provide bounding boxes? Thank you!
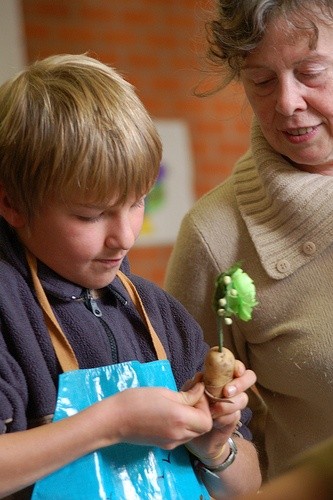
[212,260,259,352]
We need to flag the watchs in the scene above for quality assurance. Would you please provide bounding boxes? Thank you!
[196,436,238,480]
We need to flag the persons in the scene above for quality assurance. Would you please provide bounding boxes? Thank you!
[161,0,333,500]
[0,50,265,500]
